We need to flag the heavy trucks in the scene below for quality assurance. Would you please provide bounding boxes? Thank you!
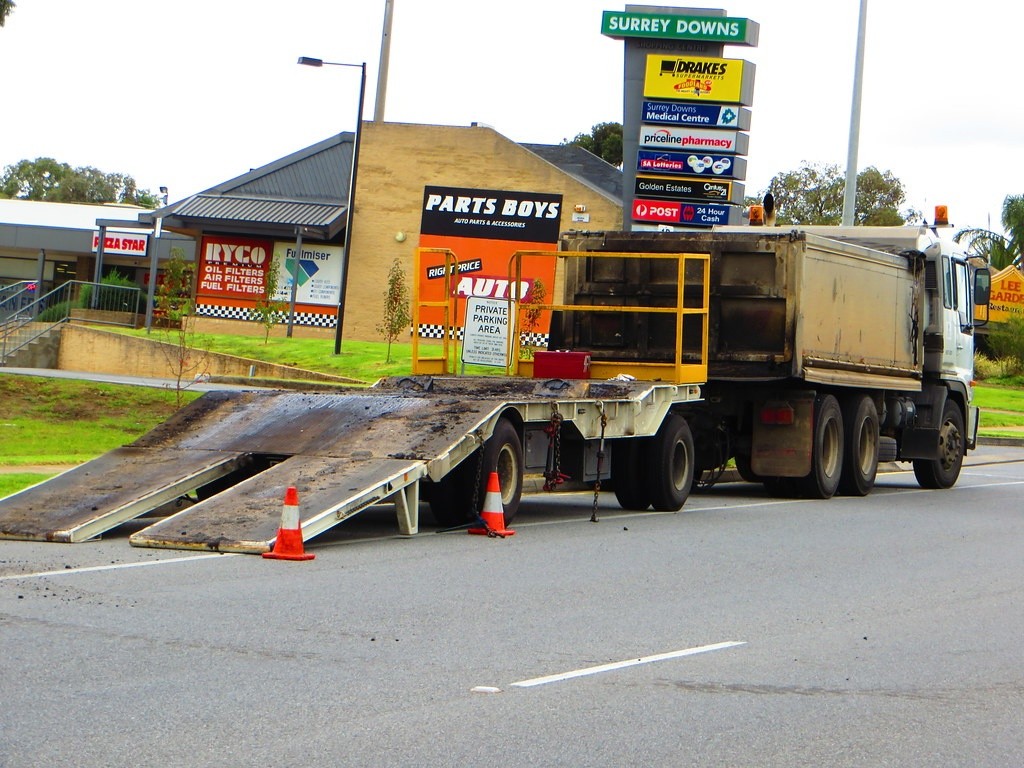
[556,190,992,556]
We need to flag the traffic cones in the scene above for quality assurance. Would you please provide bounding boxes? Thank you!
[466,472,516,536]
[261,483,316,561]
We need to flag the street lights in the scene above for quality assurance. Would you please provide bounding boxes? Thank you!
[295,56,368,355]
